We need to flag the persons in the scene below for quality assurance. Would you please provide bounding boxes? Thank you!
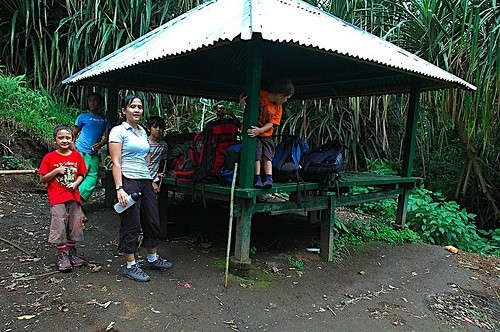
[238,75,294,187]
[114,116,168,255]
[70,92,111,221]
[108,95,173,282]
[38,125,88,271]
[206,103,242,129]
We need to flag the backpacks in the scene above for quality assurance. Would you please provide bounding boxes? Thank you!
[166,109,351,209]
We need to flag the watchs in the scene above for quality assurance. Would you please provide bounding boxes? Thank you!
[116,185,122,189]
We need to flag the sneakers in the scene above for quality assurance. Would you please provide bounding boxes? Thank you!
[69,248,82,267]
[58,251,73,271]
[144,254,173,269]
[124,264,150,282]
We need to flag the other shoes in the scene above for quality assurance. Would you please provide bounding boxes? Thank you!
[82,213,88,222]
[255,178,263,188]
[264,178,273,187]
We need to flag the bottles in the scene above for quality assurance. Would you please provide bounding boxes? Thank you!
[114,191,142,214]
